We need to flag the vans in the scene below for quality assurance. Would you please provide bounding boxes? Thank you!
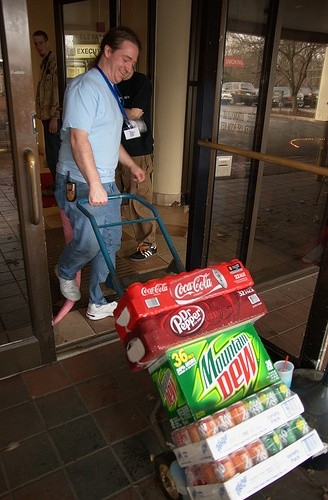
[227,82,256,107]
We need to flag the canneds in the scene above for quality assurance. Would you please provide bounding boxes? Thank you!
[184,415,310,486]
[170,381,293,448]
[168,267,227,306]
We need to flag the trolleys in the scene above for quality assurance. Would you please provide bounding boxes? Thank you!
[76,193,324,500]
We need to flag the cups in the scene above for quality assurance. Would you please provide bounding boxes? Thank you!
[273,360,294,389]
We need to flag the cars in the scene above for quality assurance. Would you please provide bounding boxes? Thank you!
[220,87,230,102]
[255,88,279,104]
[299,84,321,108]
[277,86,303,107]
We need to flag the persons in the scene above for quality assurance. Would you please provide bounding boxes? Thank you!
[115,67,158,262]
[33,30,60,181]
[53,26,145,320]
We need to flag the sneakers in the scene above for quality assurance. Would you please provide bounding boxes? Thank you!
[86,301,118,320]
[54,265,81,301]
[128,247,157,261]
